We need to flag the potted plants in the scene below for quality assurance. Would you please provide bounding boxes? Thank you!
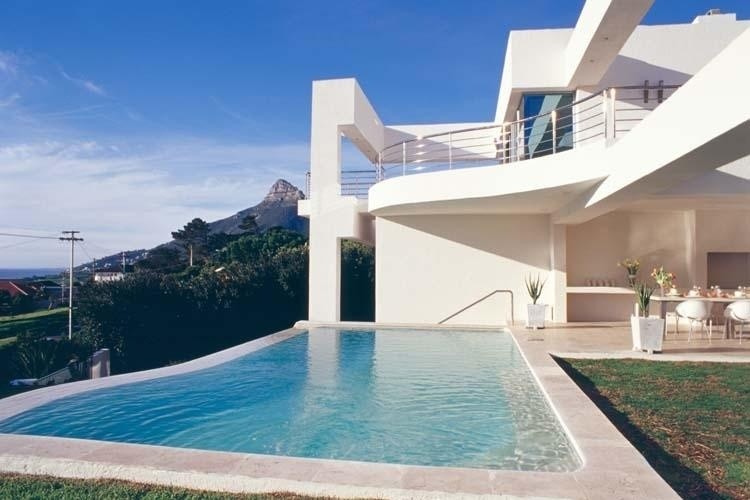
[630,281,665,355]
[525,271,545,329]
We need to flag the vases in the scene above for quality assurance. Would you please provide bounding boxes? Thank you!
[627,275,637,287]
[660,288,664,296]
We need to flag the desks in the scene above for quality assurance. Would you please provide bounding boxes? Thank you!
[639,289,750,341]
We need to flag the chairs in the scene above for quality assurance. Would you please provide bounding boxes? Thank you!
[674,300,713,344]
[722,301,750,344]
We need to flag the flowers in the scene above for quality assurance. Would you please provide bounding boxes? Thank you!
[617,256,641,275]
[651,265,676,288]
